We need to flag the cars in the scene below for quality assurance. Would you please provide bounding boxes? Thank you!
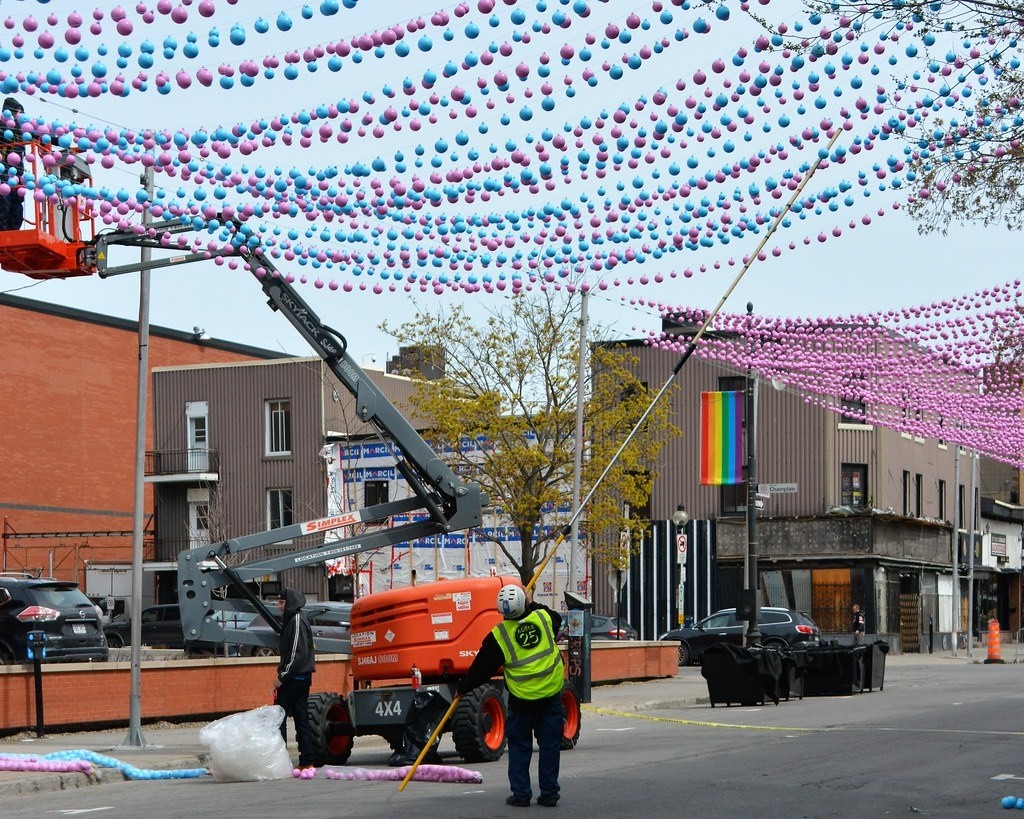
[184,606,277,657]
[103,604,184,648]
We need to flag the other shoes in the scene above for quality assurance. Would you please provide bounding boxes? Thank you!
[506,794,531,807]
[537,795,557,806]
[294,764,314,770]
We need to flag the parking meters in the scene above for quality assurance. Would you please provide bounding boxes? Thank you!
[28,631,48,738]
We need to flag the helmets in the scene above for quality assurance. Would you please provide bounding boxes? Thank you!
[496,584,527,619]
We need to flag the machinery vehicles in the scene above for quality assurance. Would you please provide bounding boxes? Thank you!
[0,136,596,770]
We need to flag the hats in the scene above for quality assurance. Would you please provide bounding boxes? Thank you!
[276,590,287,599]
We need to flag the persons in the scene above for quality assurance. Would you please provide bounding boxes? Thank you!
[453,585,565,808]
[851,604,865,645]
[269,588,317,771]
[0,97,26,231]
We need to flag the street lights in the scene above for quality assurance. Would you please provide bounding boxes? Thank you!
[673,504,688,629]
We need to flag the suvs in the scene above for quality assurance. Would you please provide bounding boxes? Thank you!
[0,573,109,665]
[658,606,820,666]
[558,612,639,641]
[239,604,351,656]
